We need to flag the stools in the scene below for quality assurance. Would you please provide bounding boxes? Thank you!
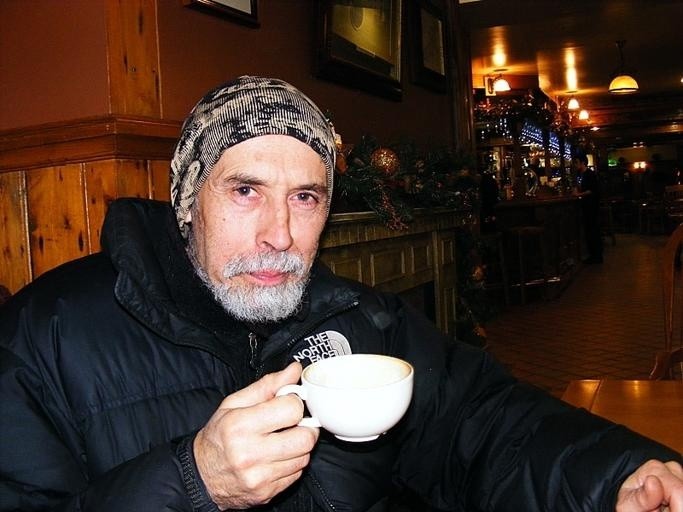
[477,225,549,307]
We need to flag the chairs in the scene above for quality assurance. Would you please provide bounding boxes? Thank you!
[647,223,683,380]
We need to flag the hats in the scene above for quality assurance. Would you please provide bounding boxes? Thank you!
[169,74,338,238]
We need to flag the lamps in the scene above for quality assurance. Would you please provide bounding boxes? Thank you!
[564,89,585,113]
[607,39,640,96]
[487,68,515,96]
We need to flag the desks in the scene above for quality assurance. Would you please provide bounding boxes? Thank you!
[562,378,683,454]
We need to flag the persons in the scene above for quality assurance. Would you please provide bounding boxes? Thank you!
[571,155,604,266]
[0,73,682,512]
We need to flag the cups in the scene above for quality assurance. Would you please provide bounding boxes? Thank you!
[274,353,414,443]
